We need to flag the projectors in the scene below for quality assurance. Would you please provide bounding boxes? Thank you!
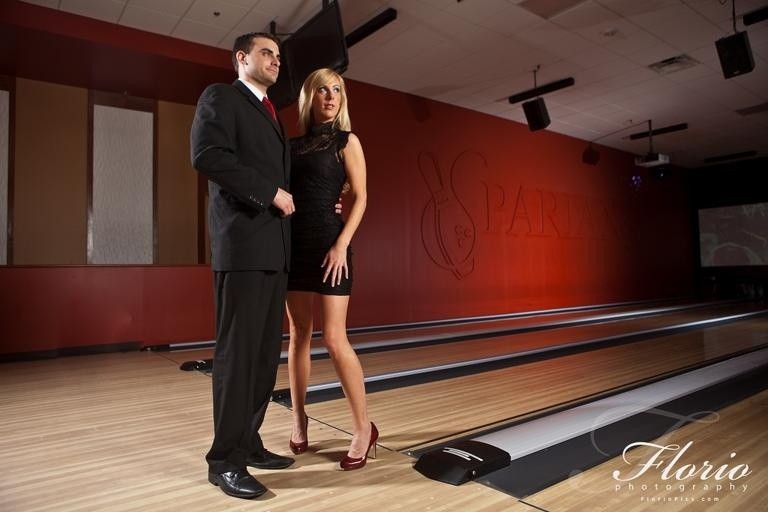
[634,152,670,167]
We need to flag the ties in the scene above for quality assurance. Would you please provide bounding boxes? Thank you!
[262,97,280,129]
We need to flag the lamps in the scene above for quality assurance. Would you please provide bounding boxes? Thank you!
[713,0,757,81]
[522,68,551,132]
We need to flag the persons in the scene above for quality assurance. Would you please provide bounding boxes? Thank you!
[284,67,379,469]
[189,32,298,499]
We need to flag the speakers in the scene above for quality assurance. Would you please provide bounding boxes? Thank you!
[522,97,551,131]
[715,30,755,80]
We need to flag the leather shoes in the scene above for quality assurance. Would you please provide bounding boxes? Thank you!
[245,448,296,469]
[208,468,268,499]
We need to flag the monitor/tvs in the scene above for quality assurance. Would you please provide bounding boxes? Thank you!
[266,0,350,112]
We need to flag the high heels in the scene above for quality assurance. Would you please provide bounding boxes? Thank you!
[289,413,308,455]
[340,422,379,470]
